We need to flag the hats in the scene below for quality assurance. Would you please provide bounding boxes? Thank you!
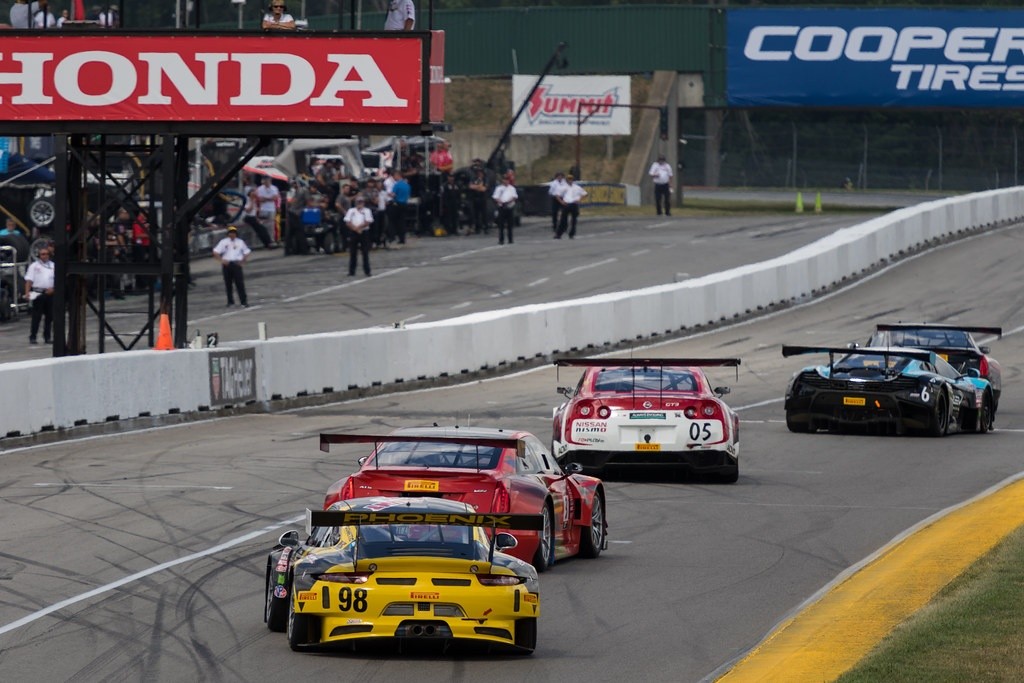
[566,174,575,178]
[355,196,365,202]
[227,226,238,232]
[502,173,511,180]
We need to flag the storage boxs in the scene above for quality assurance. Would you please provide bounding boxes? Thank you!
[302,207,321,223]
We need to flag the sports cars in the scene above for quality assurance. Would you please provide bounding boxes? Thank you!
[262,496,544,656]
[863,320,1002,431]
[320,422,610,575]
[550,356,742,483]
[780,340,977,438]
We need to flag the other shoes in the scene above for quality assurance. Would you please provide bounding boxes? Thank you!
[227,302,234,307]
[241,303,249,308]
[29,339,39,344]
[45,339,53,344]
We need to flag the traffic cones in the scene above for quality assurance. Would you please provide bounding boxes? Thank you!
[794,191,805,215]
[151,313,176,351]
[811,191,825,215]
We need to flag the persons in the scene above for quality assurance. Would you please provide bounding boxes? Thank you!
[342,197,374,277]
[213,189,238,223]
[258,177,280,217]
[648,155,672,215]
[91,6,116,26]
[548,173,588,239]
[437,172,463,235]
[87,207,151,299]
[262,0,296,29]
[465,159,490,235]
[491,175,517,245]
[212,226,251,307]
[420,142,453,172]
[0,218,55,343]
[284,141,423,254]
[240,187,280,248]
[56,9,68,28]
[383,0,415,30]
[9,0,56,27]
